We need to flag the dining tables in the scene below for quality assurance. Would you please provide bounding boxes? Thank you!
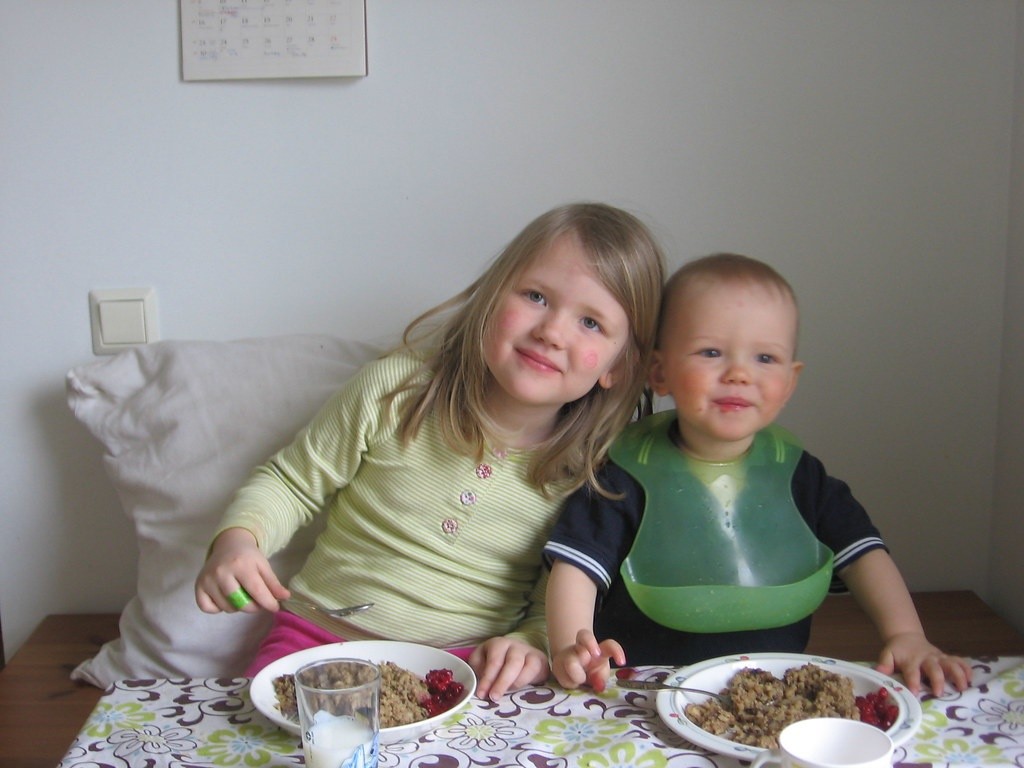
[53,651,1023,768]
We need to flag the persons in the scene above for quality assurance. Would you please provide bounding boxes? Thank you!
[195,203,662,703]
[540,255,976,699]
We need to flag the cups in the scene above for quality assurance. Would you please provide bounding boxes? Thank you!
[749,717,894,768]
[294,658,382,768]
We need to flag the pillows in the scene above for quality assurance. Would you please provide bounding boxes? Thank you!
[51,334,387,689]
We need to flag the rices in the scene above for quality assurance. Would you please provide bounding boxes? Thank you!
[684,661,861,749]
[272,660,431,728]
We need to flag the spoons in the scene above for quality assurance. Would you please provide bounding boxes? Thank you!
[277,600,375,617]
[616,680,774,709]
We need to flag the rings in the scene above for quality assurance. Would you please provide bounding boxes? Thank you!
[227,587,253,609]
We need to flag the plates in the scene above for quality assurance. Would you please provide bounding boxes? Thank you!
[656,653,922,760]
[249,640,477,743]
